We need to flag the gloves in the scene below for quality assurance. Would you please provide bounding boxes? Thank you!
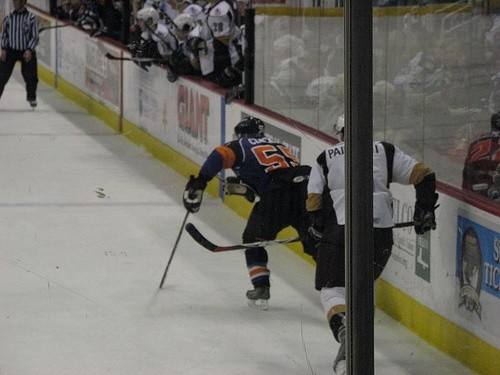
[183,177,207,213]
[222,176,254,202]
[306,223,324,244]
[413,193,439,234]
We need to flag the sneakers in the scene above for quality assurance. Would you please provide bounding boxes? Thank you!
[333,325,346,375]
[246,286,270,310]
[30,101,37,110]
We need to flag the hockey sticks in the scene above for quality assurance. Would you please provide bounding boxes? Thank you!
[37,23,73,33]
[105,52,167,63]
[159,212,190,290]
[185,212,416,252]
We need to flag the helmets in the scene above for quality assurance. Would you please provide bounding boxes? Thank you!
[137,8,160,25]
[173,14,195,31]
[234,117,265,138]
[336,114,345,132]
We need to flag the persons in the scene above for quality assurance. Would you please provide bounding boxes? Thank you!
[53,0,246,102]
[463,229,483,310]
[307,114,439,372]
[0,0,39,107]
[182,116,313,300]
